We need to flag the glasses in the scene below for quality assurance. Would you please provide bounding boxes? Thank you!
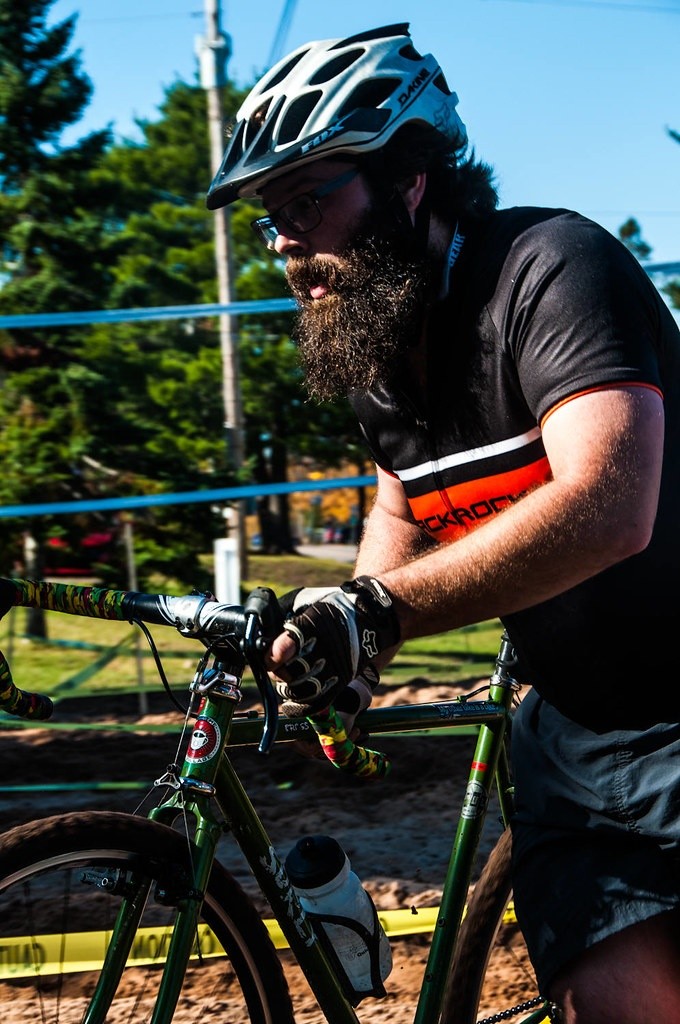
[249,158,374,258]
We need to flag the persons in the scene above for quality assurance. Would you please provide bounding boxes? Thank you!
[204,22,680,1024]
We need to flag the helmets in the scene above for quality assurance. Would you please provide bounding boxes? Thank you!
[205,22,468,211]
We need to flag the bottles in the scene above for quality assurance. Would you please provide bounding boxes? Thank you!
[284,835,392,991]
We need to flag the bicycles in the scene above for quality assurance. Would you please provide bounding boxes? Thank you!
[1,577,565,1023]
